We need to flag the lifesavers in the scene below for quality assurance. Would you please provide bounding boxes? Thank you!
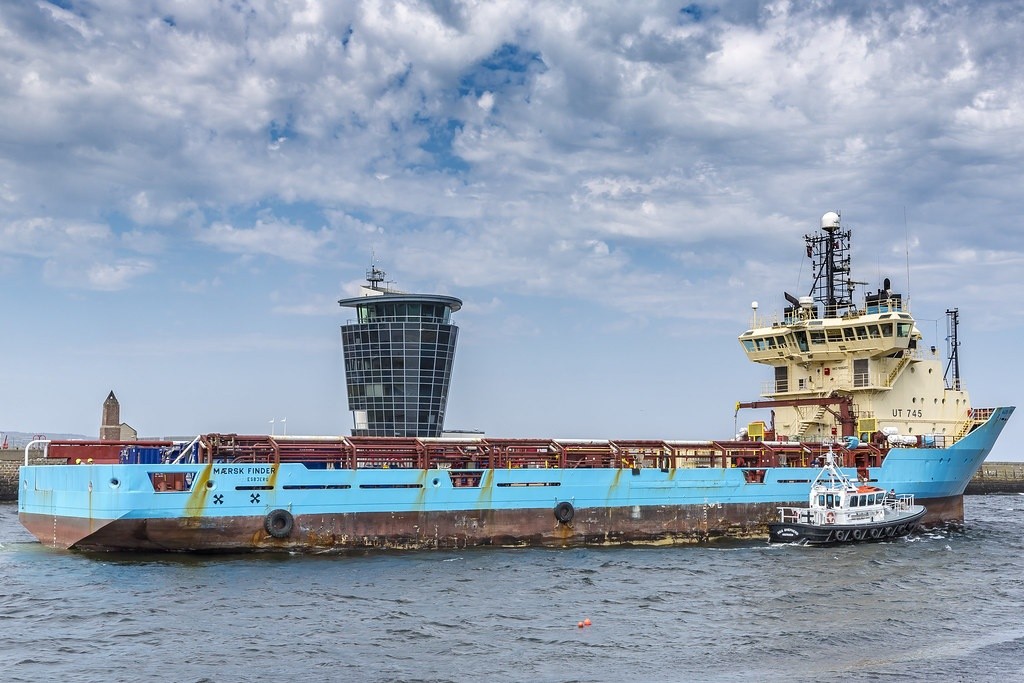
[885,527,893,536]
[827,512,834,522]
[836,530,844,540]
[898,524,906,534]
[266,509,296,538]
[853,529,862,539]
[908,523,914,532]
[871,528,878,538]
[555,502,574,522]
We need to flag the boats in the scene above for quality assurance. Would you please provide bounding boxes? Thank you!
[767,445,928,547]
[19,211,1016,554]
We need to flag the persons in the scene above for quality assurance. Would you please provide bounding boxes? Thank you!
[888,488,896,495]
